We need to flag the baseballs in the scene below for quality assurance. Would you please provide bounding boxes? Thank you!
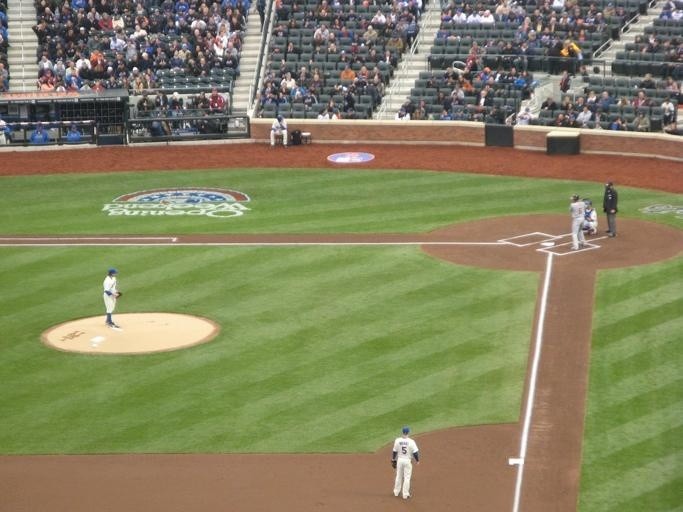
[172,237,179,241]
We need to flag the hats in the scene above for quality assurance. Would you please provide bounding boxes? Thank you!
[570,194,579,200]
[403,428,409,433]
[604,182,613,187]
[109,269,116,274]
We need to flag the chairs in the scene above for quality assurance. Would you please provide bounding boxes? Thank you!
[155,0,683,139]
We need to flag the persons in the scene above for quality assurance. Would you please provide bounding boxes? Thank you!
[604,182,618,239]
[569,192,589,250]
[391,426,420,500]
[1,1,683,150]
[103,269,120,329]
[583,198,600,237]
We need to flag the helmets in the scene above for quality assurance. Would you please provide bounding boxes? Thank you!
[583,199,592,205]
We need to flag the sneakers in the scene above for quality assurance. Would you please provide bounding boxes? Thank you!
[109,323,119,328]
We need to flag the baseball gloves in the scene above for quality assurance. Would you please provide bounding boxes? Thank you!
[391,459,397,468]
